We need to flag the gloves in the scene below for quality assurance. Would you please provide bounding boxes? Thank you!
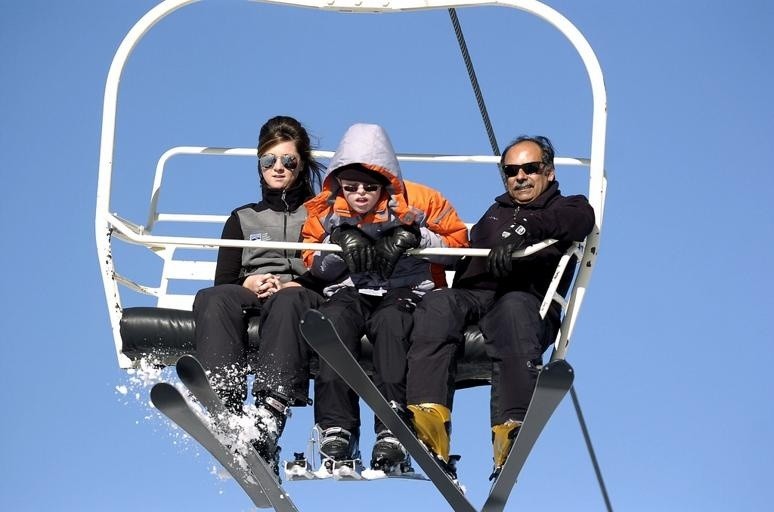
[485,220,532,279]
[329,220,421,283]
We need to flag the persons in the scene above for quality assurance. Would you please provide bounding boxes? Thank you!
[191,115,327,460]
[388,134,596,479]
[302,123,470,470]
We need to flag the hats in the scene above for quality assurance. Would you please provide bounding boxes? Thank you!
[335,161,388,185]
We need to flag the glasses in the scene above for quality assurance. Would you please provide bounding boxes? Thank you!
[501,161,551,177]
[259,152,299,170]
[343,185,378,192]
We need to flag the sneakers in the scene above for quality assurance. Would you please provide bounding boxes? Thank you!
[207,363,290,475]
[318,426,362,471]
[370,430,414,475]
[391,399,451,464]
[490,420,522,477]
